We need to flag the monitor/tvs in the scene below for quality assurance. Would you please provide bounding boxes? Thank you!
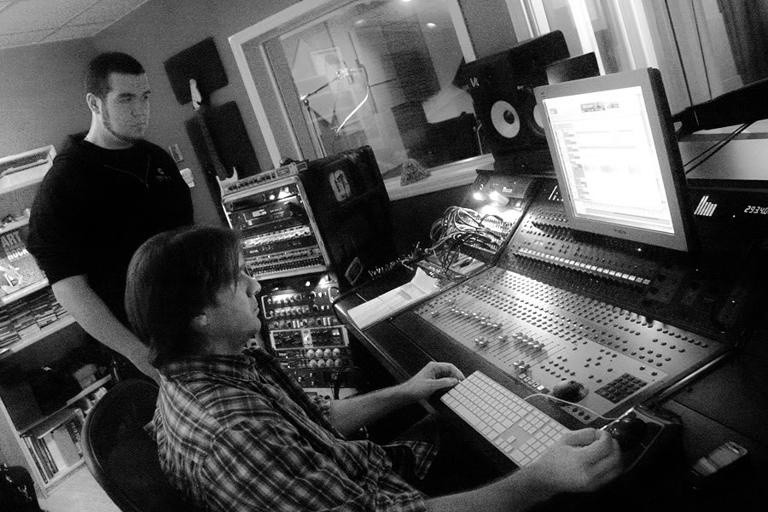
[532,69,697,253]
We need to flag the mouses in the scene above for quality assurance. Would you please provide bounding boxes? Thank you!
[608,416,646,452]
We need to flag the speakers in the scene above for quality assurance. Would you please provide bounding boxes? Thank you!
[461,29,570,163]
[539,53,601,85]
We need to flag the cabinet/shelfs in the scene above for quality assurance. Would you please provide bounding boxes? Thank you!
[0,143,119,501]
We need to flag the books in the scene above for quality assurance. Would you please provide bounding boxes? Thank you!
[1,228,47,298]
[21,387,110,485]
[348,267,443,330]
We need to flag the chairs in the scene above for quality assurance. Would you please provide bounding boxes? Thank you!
[80,380,210,512]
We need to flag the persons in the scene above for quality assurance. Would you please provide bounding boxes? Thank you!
[25,51,194,391]
[124,226,625,510]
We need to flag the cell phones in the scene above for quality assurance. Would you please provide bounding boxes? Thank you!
[693,440,748,478]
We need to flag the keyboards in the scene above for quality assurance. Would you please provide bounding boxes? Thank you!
[440,370,574,467]
